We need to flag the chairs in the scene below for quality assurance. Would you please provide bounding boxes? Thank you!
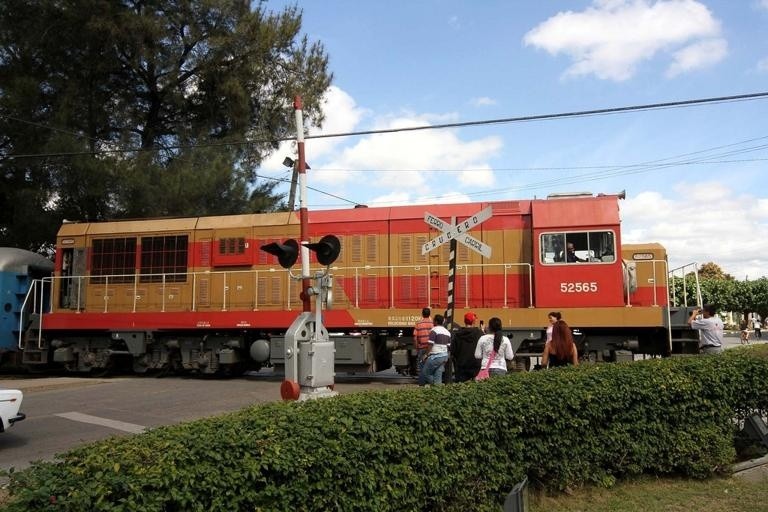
[554,247,559,262]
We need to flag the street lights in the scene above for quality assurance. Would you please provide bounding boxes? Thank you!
[281,157,309,211]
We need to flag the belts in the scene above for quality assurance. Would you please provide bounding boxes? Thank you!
[704,344,721,348]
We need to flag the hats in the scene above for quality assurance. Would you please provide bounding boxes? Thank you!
[464,311,478,323]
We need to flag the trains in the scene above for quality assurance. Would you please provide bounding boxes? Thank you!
[0,187,704,379]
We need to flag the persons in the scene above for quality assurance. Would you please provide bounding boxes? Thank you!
[545,311,563,370]
[473,317,514,379]
[541,320,578,369]
[753,319,763,337]
[449,312,486,384]
[740,319,749,340]
[412,308,435,387]
[419,314,452,388]
[688,303,724,354]
[562,242,586,263]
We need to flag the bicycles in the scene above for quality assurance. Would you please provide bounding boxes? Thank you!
[740,329,751,345]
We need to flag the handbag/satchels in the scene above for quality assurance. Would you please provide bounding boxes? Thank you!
[475,369,490,382]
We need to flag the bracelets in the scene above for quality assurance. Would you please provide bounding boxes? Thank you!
[424,354,428,358]
